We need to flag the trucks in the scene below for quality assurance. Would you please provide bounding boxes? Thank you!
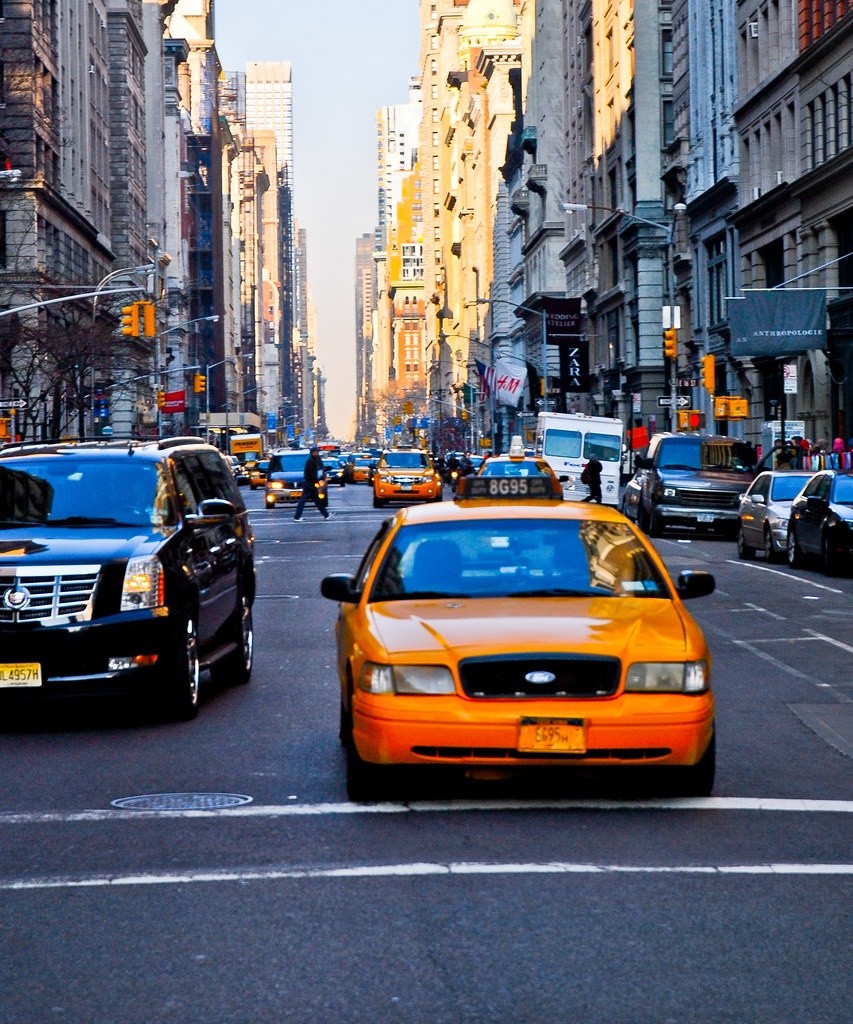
[229,434,264,463]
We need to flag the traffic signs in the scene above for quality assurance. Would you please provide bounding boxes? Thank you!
[515,398,556,418]
[0,398,30,409]
[656,395,691,408]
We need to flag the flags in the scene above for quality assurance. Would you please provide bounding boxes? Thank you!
[161,390,185,414]
[542,295,581,344]
[723,289,828,356]
[463,358,527,407]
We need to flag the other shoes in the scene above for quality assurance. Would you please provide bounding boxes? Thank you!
[326,512,336,520]
[294,516,306,522]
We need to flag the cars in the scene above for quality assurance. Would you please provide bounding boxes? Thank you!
[319,498,716,803]
[369,445,443,509]
[433,447,570,501]
[222,448,279,491]
[309,442,383,488]
[621,431,853,579]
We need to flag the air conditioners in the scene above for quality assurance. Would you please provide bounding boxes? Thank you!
[572,108,576,116]
[103,139,110,148]
[576,35,581,44]
[747,20,762,40]
[88,64,95,74]
[581,39,586,44]
[571,47,576,55]
[100,19,107,28]
[774,170,784,186]
[576,100,582,109]
[104,199,110,209]
[579,136,584,143]
[751,186,763,200]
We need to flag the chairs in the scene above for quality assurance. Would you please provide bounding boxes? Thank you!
[404,539,471,595]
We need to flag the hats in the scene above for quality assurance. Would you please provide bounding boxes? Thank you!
[589,453,596,460]
[310,447,321,454]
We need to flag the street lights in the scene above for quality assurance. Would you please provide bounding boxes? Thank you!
[90,264,157,440]
[225,372,300,455]
[560,200,681,430]
[428,298,551,456]
[206,353,253,445]
[157,314,220,435]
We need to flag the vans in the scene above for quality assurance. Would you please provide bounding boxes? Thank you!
[534,412,624,504]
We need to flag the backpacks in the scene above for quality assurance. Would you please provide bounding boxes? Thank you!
[580,463,591,485]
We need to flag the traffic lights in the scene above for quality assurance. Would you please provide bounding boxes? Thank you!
[122,303,138,336]
[194,374,206,393]
[157,390,166,407]
[662,327,715,430]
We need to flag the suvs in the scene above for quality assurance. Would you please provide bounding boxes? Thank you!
[0,436,258,724]
[259,448,333,509]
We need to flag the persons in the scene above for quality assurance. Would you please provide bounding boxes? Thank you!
[294,447,336,522]
[747,436,844,471]
[580,454,602,504]
[634,451,644,470]
[432,450,492,492]
